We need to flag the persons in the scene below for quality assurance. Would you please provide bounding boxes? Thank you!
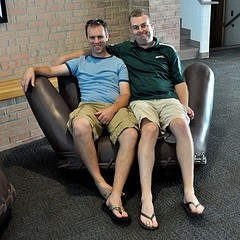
[53,8,205,231]
[21,19,140,223]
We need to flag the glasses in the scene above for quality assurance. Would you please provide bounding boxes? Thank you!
[86,19,104,25]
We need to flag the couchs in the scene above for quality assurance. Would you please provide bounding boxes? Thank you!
[24,62,215,171]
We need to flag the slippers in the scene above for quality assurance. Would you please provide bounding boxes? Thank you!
[101,203,132,223]
[139,212,160,230]
[181,202,206,216]
[121,192,129,201]
